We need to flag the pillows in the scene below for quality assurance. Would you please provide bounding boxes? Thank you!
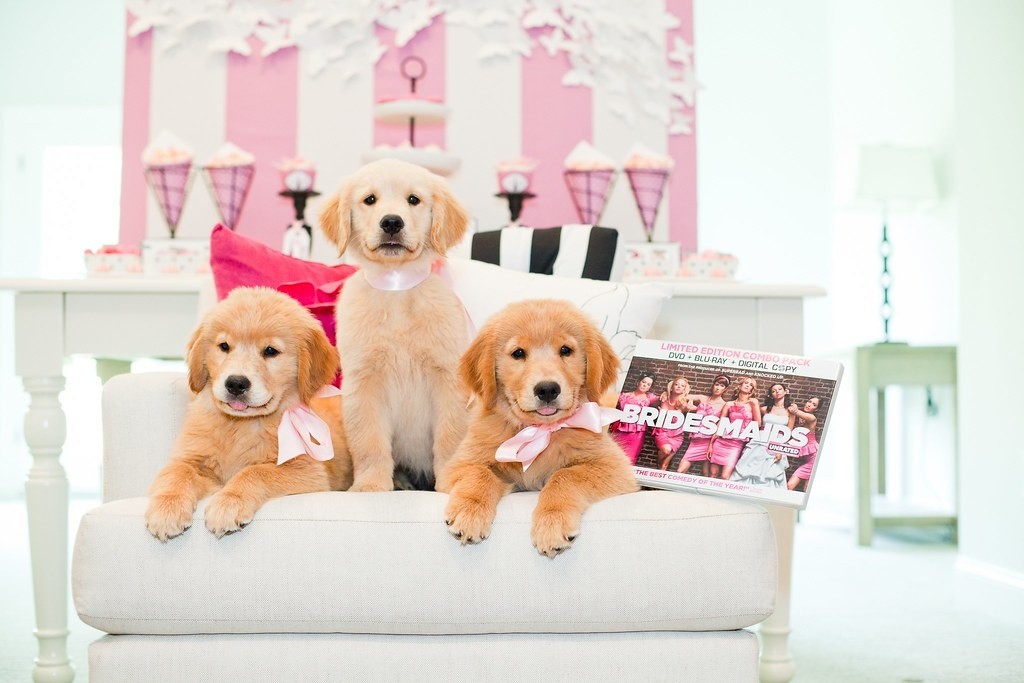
[435,258,678,391]
[209,221,359,388]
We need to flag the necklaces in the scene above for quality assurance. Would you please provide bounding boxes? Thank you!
[669,400,674,406]
[635,391,637,396]
[708,397,714,404]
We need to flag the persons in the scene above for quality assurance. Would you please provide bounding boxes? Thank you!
[610,372,823,492]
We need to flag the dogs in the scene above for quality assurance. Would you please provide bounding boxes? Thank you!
[319,158,476,491]
[442,299,641,561]
[144,284,413,543]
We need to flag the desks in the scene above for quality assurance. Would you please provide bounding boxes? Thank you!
[0,275,830,683]
[72,370,780,683]
[853,343,958,547]
